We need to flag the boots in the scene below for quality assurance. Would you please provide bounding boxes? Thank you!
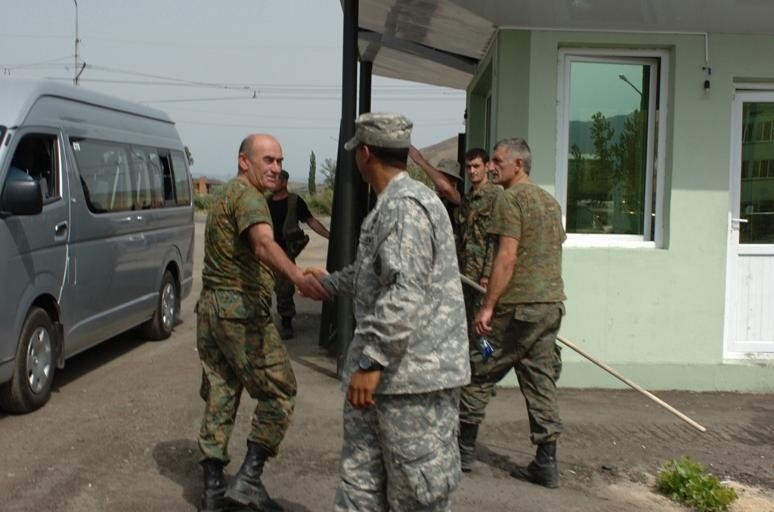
[225,443,284,512]
[510,442,558,488]
[203,462,228,512]
[458,423,478,471]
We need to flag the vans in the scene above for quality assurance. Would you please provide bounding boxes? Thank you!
[1,79,195,411]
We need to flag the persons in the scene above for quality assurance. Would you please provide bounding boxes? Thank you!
[458,148,504,343]
[297,112,471,512]
[266,170,330,340]
[408,144,464,234]
[195,134,332,512]
[457,137,567,488]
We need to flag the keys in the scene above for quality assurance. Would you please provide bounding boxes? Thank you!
[479,335,494,360]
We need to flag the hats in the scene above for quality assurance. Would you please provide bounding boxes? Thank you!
[435,156,465,184]
[344,113,412,151]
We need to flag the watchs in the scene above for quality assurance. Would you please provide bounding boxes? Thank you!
[356,352,386,372]
